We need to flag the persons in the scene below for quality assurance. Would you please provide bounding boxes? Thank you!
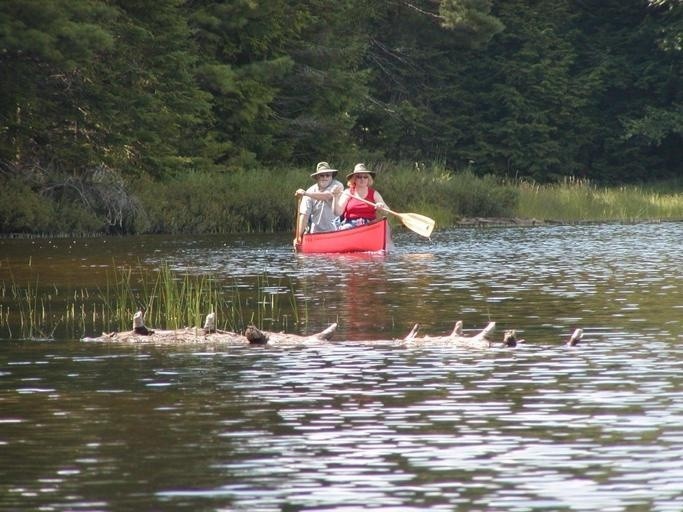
[330,161,390,231]
[291,161,344,246]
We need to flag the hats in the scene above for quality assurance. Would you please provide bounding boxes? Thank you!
[310,161,338,179]
[344,162,376,180]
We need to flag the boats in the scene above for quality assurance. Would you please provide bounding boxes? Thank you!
[297,215,391,259]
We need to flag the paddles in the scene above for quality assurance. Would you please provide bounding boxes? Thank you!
[340,191,436,239]
